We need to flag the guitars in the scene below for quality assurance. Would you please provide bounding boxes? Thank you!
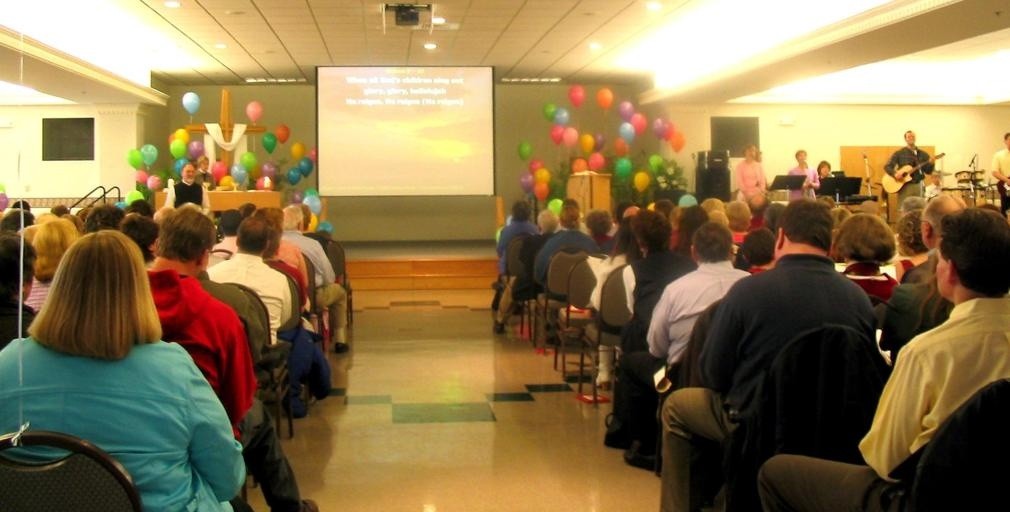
[883,153,946,193]
[996,176,1010,196]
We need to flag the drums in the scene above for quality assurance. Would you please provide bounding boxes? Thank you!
[955,171,973,184]
[973,171,985,184]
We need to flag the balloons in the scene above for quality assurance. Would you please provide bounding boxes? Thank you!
[545,85,673,146]
[127,92,317,168]
[515,141,699,216]
[115,154,162,210]
[175,155,321,215]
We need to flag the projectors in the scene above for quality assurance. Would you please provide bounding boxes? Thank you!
[396,10,418,25]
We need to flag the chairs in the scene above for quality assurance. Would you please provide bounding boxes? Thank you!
[0,196,351,512]
[496,222,1008,512]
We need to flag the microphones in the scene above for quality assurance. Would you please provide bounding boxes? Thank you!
[969,154,977,167]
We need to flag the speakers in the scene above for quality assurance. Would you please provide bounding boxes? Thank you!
[696,168,731,205]
[698,151,729,170]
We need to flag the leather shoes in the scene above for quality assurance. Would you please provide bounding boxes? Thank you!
[335,342,348,352]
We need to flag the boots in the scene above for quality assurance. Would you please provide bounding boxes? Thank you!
[595,344,615,391]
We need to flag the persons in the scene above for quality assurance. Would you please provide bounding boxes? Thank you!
[787,149,832,202]
[194,155,217,191]
[736,144,768,197]
[992,131,1009,218]
[884,130,935,212]
[2,198,348,512]
[164,162,211,216]
[490,185,1009,512]
